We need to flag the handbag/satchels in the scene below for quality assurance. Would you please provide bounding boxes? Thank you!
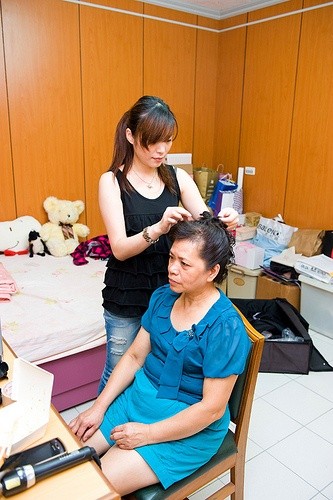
[193,162,333,289]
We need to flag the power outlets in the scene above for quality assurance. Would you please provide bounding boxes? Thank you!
[245,166,255,175]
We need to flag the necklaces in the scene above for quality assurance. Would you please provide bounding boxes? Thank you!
[131,167,156,188]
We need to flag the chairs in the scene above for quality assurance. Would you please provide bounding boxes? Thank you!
[121,303,265,500]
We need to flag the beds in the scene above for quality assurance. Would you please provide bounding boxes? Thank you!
[0,252,108,412]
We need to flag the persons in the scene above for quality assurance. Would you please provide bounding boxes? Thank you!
[97,96,239,399]
[68,211,253,497]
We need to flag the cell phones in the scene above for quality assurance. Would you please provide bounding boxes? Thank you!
[5,438,65,469]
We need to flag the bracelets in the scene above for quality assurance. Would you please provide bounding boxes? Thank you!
[142,226,159,244]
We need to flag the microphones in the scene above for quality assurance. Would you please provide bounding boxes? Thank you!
[0,446,97,497]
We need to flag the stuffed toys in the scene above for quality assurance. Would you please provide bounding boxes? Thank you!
[0,196,90,257]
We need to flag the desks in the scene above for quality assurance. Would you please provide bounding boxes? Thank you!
[0,334,119,500]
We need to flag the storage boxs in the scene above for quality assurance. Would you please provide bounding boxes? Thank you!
[228,298,312,375]
[231,242,265,270]
[298,273,333,339]
[255,271,302,310]
[1,357,54,459]
[225,264,264,299]
